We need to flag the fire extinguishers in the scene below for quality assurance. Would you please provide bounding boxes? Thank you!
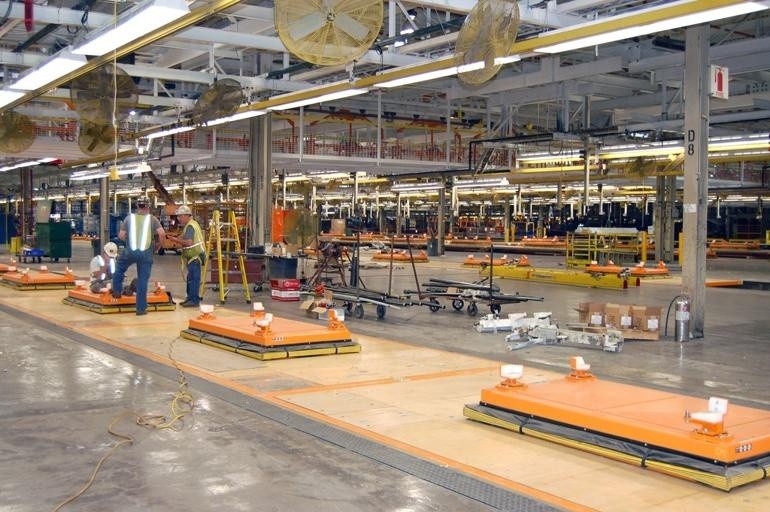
[665,295,690,343]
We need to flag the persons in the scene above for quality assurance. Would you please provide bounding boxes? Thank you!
[167,206,206,307]
[112,196,165,315]
[90,242,117,293]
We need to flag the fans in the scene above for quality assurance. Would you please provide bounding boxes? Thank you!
[0,110,38,154]
[453,0,521,87]
[274,0,384,66]
[191,77,243,132]
[70,61,139,157]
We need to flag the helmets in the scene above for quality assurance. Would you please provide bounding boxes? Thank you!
[104,242,118,258]
[136,196,150,205]
[175,205,192,216]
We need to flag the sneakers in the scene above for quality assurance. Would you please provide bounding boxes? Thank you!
[136,310,147,315]
[180,300,199,307]
[112,294,122,299]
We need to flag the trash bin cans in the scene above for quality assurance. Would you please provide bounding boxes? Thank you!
[91,239,101,256]
[11,238,22,253]
[427,239,438,256]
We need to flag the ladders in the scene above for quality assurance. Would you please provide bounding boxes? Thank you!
[199,207,252,305]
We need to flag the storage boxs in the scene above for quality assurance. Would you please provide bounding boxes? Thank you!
[578,299,665,343]
